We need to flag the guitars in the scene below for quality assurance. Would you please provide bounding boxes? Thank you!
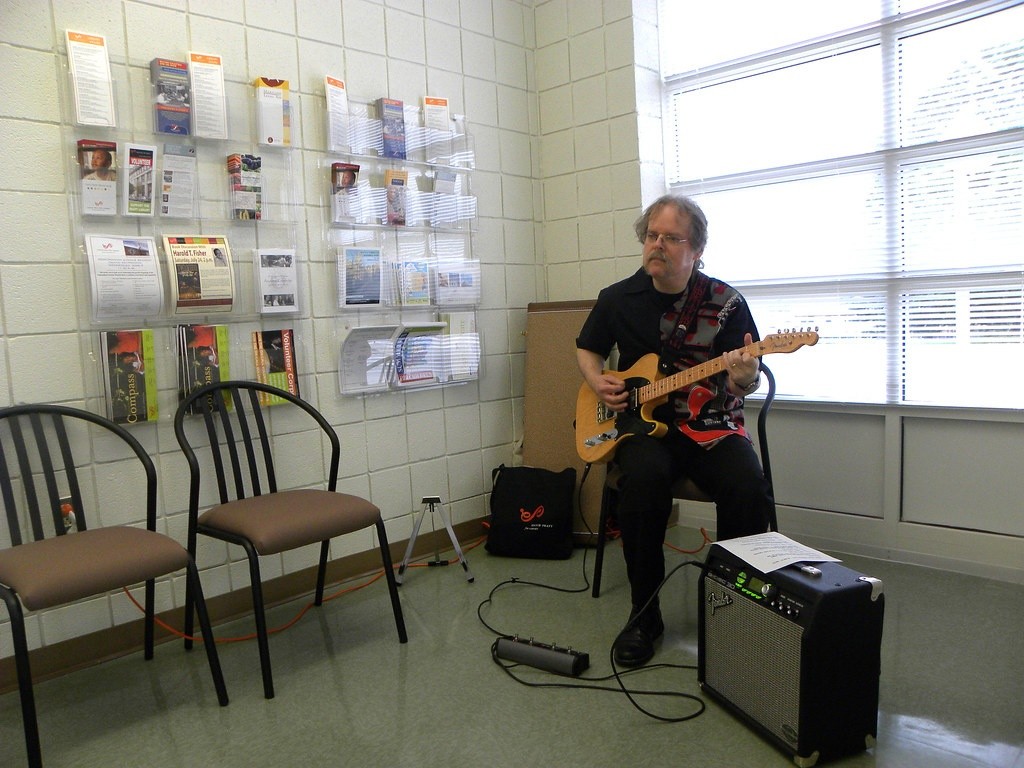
[575,326,819,465]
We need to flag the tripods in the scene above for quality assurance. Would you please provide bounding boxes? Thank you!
[394,496,475,586]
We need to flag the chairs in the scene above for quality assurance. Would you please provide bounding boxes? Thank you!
[0,405,232,767]
[172,381,408,700]
[592,364,781,603]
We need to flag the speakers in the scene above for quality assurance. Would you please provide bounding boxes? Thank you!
[694,546,886,768]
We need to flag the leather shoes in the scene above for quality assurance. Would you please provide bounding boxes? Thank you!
[614,605,665,668]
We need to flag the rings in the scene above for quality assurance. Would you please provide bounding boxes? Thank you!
[732,364,735,366]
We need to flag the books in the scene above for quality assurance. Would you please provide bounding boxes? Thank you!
[98,328,160,426]
[336,245,383,310]
[331,162,362,224]
[375,97,407,160]
[432,170,457,196]
[385,169,408,226]
[150,57,193,136]
[175,323,233,414]
[252,249,299,313]
[254,77,291,146]
[438,312,479,381]
[226,153,261,220]
[392,260,430,306]
[252,328,300,408]
[76,139,117,216]
[121,142,158,218]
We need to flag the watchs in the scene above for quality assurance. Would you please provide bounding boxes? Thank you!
[734,374,761,391]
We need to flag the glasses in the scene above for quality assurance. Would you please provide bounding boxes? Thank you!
[643,233,691,247]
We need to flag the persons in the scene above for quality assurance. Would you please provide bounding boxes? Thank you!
[575,196,775,669]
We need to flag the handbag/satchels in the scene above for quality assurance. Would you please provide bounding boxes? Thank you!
[486,462,577,561]
